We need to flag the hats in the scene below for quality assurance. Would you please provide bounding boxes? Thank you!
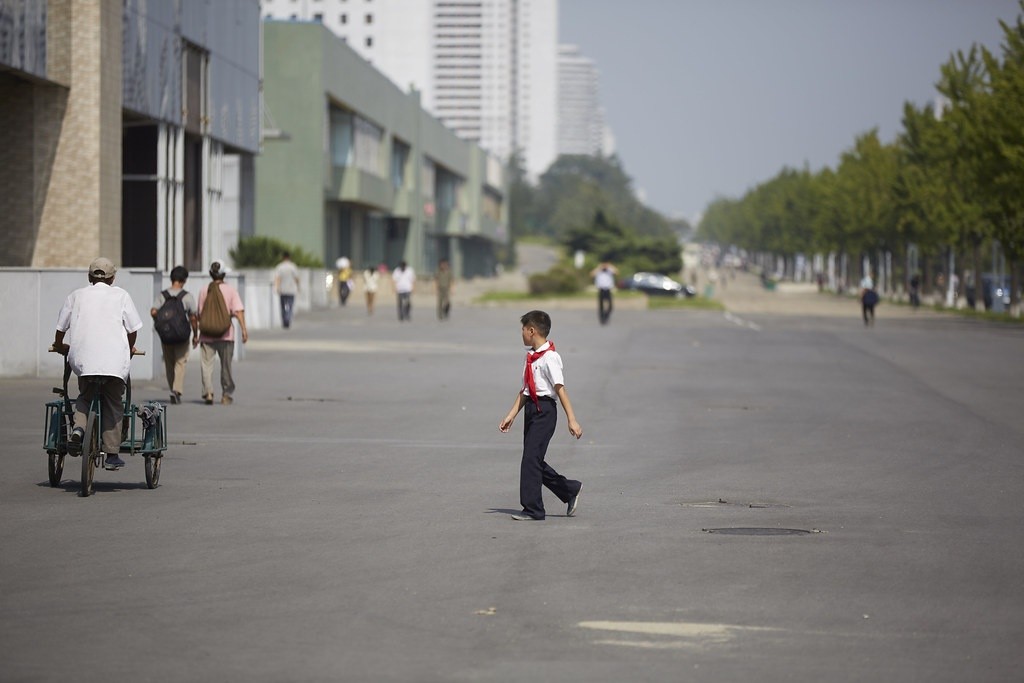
[89,257,117,279]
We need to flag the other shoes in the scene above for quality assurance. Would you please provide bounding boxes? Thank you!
[511,512,536,521]
[205,394,213,405]
[222,397,231,406]
[105,453,125,470]
[68,429,83,457]
[169,391,180,405]
[567,483,583,516]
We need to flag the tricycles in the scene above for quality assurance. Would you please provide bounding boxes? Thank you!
[44,345,169,498]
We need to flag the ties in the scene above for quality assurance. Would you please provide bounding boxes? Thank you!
[522,342,556,413]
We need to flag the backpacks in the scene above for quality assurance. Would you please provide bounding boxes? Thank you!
[154,290,191,344]
[199,282,231,338]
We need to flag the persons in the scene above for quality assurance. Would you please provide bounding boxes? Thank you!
[197,260,247,405]
[392,261,416,322]
[909,273,920,305]
[435,257,453,319]
[499,311,583,520]
[52,257,143,470]
[860,274,876,323]
[338,267,353,305]
[364,267,378,309]
[595,267,614,324]
[273,251,299,329]
[152,265,199,404]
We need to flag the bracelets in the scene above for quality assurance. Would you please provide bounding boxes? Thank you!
[52,343,64,349]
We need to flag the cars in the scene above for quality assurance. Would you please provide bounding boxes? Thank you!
[618,271,699,304]
